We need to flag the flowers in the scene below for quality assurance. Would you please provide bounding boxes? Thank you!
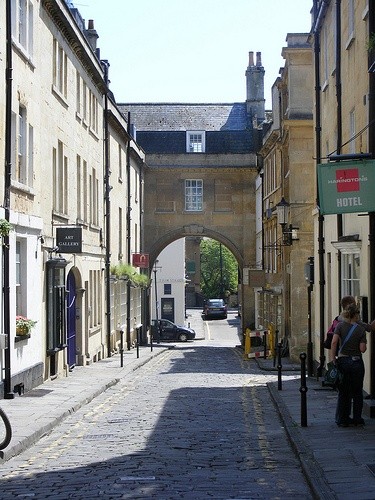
[16,315,36,329]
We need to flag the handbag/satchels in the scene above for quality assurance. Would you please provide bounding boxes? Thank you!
[322,332,333,349]
[324,360,339,382]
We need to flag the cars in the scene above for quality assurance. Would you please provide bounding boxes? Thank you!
[151,319,195,342]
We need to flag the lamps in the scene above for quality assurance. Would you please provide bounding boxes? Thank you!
[276,198,290,233]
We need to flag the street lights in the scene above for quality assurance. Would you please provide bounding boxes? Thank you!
[152,259,161,343]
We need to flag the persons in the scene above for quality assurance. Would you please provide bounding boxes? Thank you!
[324,296,375,426]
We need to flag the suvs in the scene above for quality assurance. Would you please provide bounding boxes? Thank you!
[202,298,228,319]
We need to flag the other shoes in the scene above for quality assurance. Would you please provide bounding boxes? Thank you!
[338,417,351,427]
[351,417,364,428]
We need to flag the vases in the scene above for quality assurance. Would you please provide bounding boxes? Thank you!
[17,328,28,336]
